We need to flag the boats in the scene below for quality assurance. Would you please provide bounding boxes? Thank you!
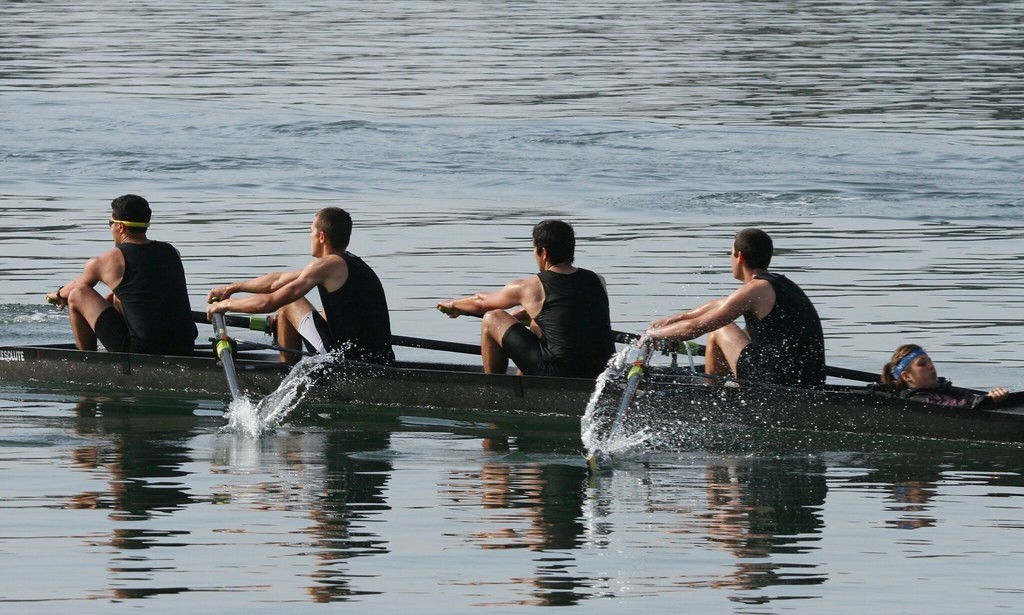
[1,292,1023,477]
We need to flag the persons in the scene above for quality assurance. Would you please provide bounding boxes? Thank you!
[880,344,1009,407]
[58,393,958,612]
[638,229,825,389]
[206,208,395,363]
[436,220,616,380]
[46,194,198,353]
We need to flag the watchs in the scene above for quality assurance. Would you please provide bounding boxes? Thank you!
[56,285,66,299]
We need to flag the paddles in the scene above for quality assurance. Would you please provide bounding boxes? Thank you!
[204,299,248,404]
[662,331,881,386]
[437,296,709,357]
[586,322,653,481]
[49,291,275,336]
[387,334,484,357]
